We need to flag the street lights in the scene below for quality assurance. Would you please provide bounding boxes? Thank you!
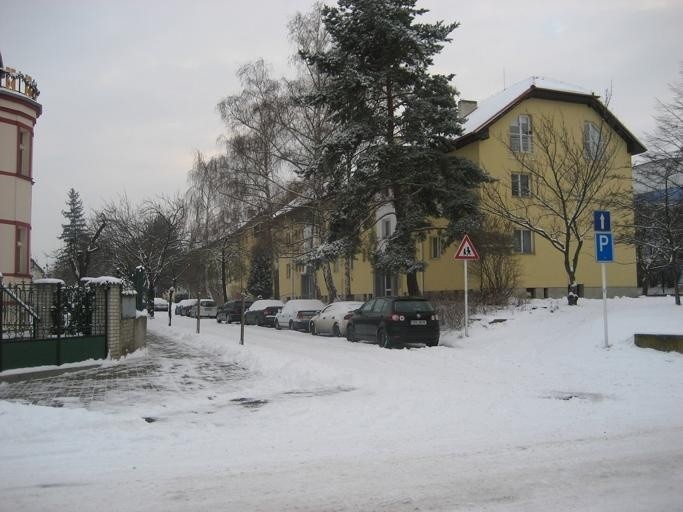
[195,291,201,334]
[237,288,247,346]
[166,286,174,326]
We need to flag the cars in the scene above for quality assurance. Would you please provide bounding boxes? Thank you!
[306,300,368,338]
[174,294,330,332]
[152,296,169,312]
[343,295,441,349]
